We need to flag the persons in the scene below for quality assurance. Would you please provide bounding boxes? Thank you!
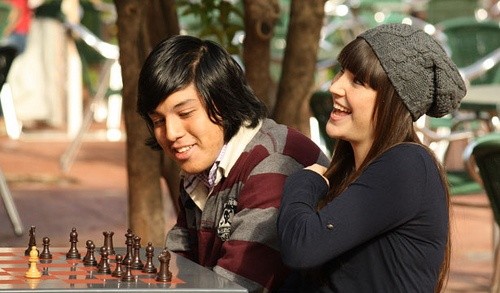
[136,34,333,293]
[277,24,467,293]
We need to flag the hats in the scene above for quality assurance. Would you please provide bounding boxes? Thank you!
[357,23,467,121]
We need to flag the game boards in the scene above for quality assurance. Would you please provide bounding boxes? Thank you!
[0,250,186,290]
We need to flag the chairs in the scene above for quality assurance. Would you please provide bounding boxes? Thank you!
[462,133,500,293]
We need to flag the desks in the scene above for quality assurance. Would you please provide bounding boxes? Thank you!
[0,247,248,293]
